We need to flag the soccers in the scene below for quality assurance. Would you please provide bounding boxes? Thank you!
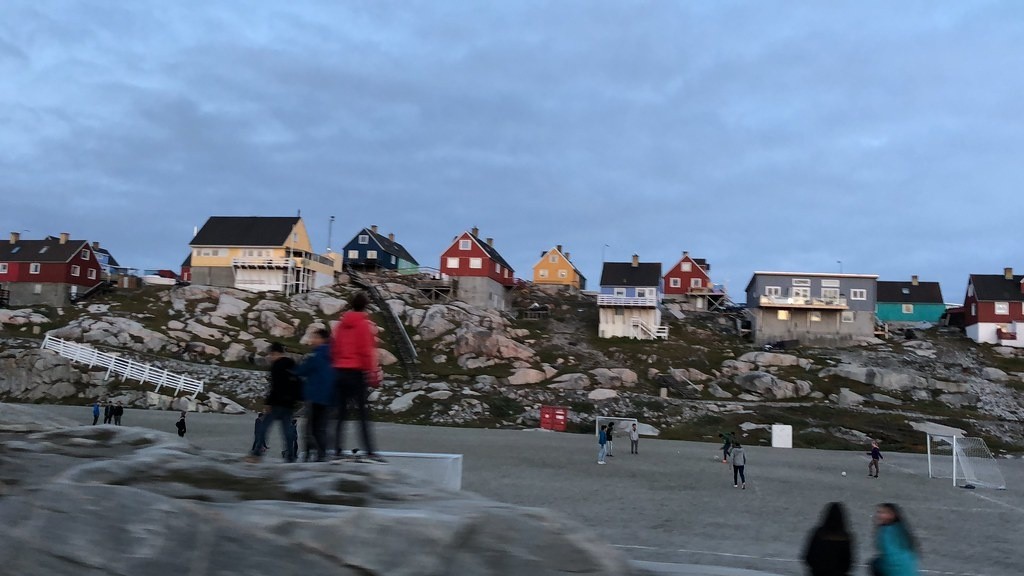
[841,471,847,477]
[713,455,720,461]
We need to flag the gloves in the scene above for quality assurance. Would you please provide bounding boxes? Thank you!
[365,369,379,387]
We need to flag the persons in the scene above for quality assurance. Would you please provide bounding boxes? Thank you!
[113,401,124,426]
[92,400,101,426]
[597,422,614,464]
[867,503,922,576]
[866,440,883,479]
[104,401,114,424]
[629,423,639,455]
[718,431,746,489]
[799,501,858,576]
[176,410,187,437]
[248,328,333,463]
[332,292,390,463]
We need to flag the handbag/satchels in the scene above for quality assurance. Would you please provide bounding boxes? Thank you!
[175,421,180,428]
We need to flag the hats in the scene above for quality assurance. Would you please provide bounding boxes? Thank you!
[299,322,330,344]
[259,413,264,417]
[271,341,285,354]
[181,410,187,417]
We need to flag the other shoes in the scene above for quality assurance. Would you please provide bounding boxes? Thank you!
[597,460,607,465]
[742,482,746,489]
[733,484,737,487]
[358,452,391,465]
[722,460,727,464]
[329,454,353,464]
